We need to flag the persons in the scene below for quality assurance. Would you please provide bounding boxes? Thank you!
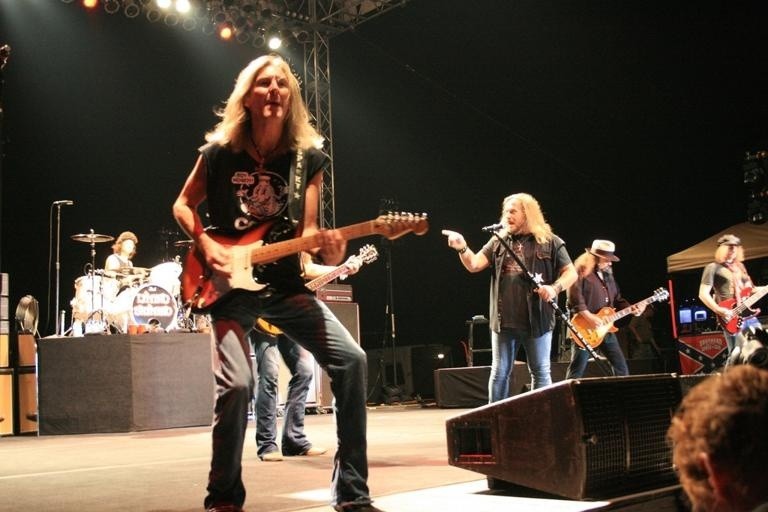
[666,365,767,511]
[442,192,579,404]
[172,54,384,511]
[697,234,768,372]
[248,257,361,462]
[102,231,139,334]
[565,238,646,378]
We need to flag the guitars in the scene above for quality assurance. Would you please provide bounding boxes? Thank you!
[570,287,670,349]
[181,211,430,315]
[717,287,768,334]
[253,243,379,337]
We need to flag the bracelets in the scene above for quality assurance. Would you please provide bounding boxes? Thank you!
[457,245,467,254]
[551,281,563,294]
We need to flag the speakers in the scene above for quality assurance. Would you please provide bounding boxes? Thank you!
[444,373,722,501]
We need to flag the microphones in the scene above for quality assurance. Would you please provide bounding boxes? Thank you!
[480,221,504,233]
[54,199,73,205]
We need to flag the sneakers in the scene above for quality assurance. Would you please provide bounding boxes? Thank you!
[304,446,327,456]
[262,450,284,461]
[333,503,383,512]
[207,500,244,511]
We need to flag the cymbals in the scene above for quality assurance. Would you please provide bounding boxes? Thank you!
[173,240,195,248]
[71,234,114,243]
[116,267,150,276]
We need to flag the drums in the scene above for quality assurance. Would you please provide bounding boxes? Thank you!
[70,275,123,334]
[118,284,177,333]
[149,262,183,297]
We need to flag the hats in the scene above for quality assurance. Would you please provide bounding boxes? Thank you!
[716,234,742,246]
[584,239,621,262]
